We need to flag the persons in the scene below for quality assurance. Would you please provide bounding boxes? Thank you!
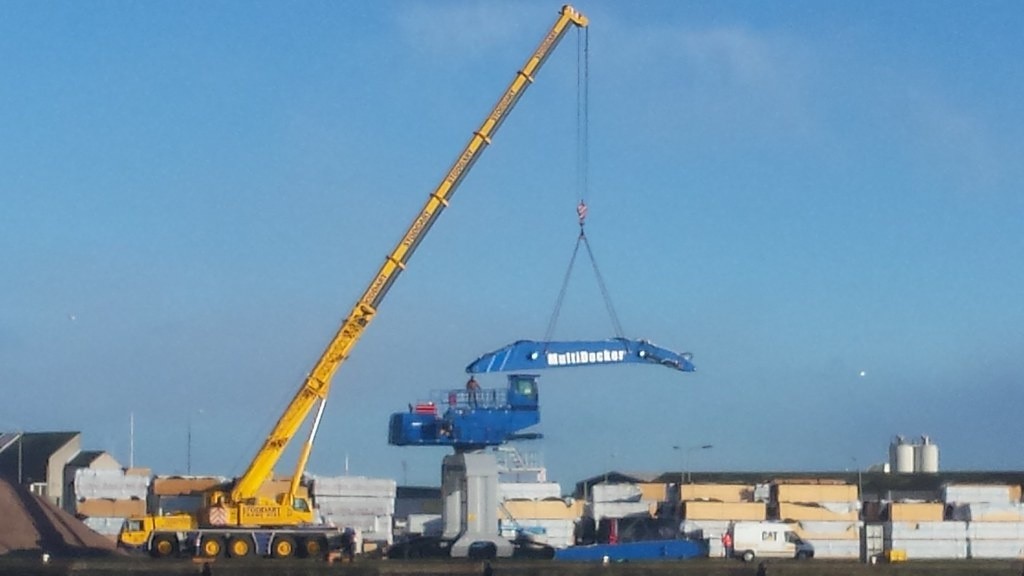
[723,532,733,559]
[465,376,482,409]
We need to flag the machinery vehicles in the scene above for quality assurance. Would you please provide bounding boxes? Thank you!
[380,337,697,454]
[112,5,590,561]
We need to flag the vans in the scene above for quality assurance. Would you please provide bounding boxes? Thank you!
[732,522,815,563]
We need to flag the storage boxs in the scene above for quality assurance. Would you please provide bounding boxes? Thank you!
[500,481,1024,561]
[62,466,397,545]
